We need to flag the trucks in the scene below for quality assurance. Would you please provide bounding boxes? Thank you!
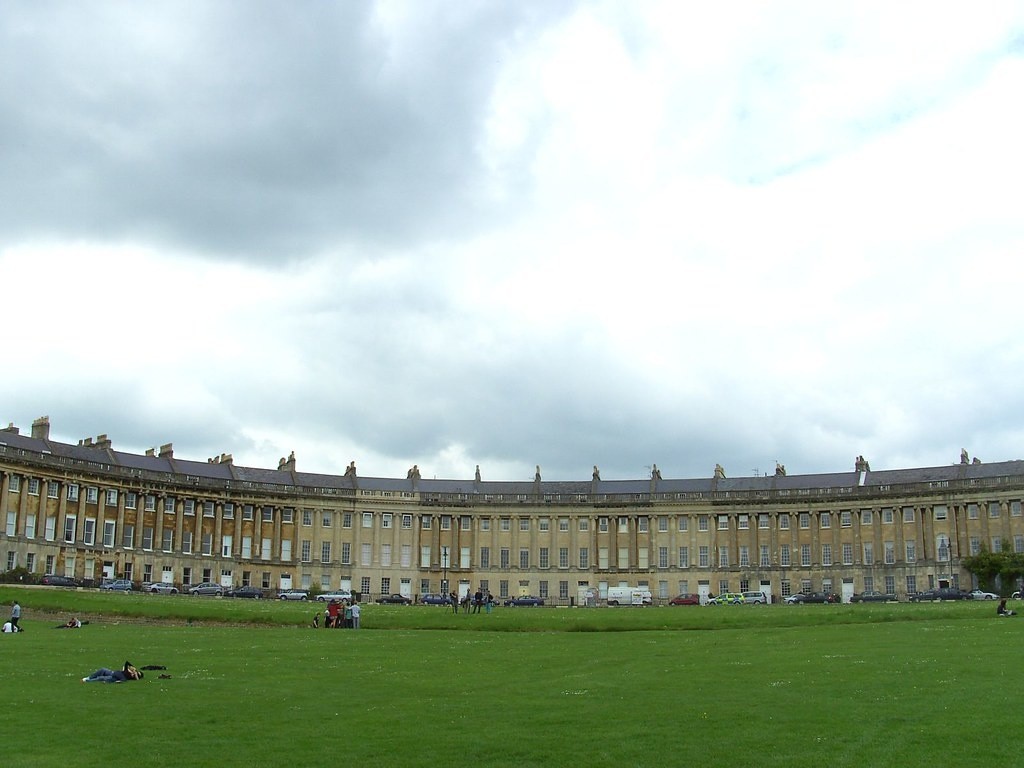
[607,586,652,606]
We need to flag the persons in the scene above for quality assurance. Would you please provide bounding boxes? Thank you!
[313,613,320,628]
[451,589,458,614]
[11,601,22,632]
[464,588,471,614]
[485,591,493,614]
[473,588,483,613]
[82,661,144,682]
[67,617,81,628]
[325,598,360,629]
[2,621,12,632]
[997,600,1007,614]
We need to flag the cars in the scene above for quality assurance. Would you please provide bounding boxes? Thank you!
[420,593,453,606]
[375,594,412,605]
[909,587,1000,603]
[504,595,545,607]
[784,592,839,605]
[41,575,80,587]
[669,593,700,606]
[140,582,179,596]
[314,590,352,604]
[224,586,264,600]
[187,582,224,597]
[461,595,500,607]
[850,590,898,604]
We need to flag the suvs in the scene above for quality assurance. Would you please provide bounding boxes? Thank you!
[99,579,132,594]
[705,590,767,605]
[276,589,310,602]
